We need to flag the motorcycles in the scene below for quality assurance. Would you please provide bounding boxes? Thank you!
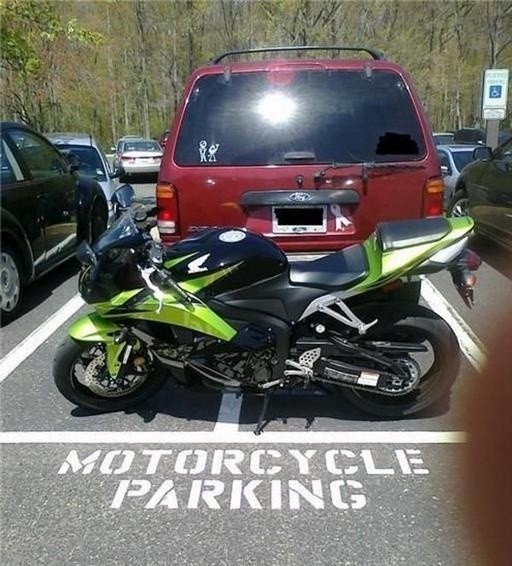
[50,182,484,436]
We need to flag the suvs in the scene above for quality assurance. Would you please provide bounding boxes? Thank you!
[154,44,447,310]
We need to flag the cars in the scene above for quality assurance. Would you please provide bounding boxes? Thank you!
[0,118,109,322]
[432,131,455,146]
[114,133,164,181]
[445,134,512,256]
[16,128,126,232]
[435,142,492,218]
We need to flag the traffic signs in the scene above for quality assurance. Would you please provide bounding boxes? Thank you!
[480,69,508,120]
